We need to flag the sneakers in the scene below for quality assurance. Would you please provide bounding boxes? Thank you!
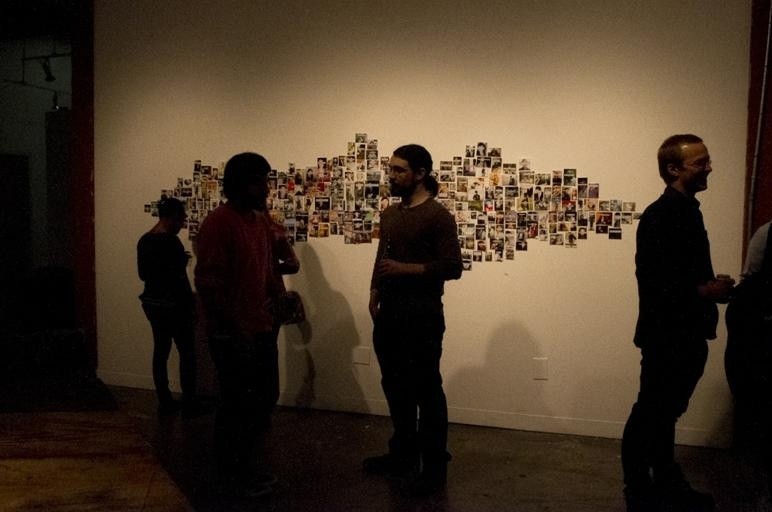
[624,469,715,511]
[365,439,452,506]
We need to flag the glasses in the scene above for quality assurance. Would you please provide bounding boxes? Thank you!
[679,160,712,171]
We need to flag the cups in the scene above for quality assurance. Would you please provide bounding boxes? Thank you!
[715,274,730,282]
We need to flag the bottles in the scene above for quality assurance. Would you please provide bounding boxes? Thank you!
[382,239,392,260]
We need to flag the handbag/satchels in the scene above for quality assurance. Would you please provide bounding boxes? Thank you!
[272,272,306,326]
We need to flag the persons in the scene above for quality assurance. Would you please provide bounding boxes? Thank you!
[193,151,281,503]
[135,192,195,428]
[616,131,738,512]
[365,142,464,495]
[266,207,302,411]
[432,143,642,268]
[268,137,400,247]
[146,160,224,241]
[722,220,772,512]
